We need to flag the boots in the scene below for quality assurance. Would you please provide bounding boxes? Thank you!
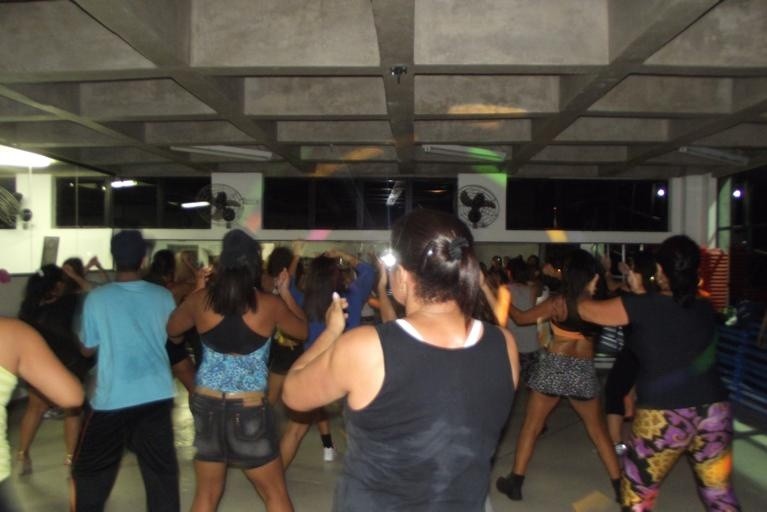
[611,479,622,503]
[496,471,526,500]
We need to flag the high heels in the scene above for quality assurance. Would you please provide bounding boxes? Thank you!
[16,450,32,475]
[63,454,74,478]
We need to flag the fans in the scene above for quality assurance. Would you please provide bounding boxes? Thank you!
[451,184,500,231]
[196,183,245,228]
[0,187,32,226]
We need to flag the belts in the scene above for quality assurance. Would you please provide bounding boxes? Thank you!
[190,384,267,401]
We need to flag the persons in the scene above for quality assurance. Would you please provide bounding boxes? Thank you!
[475,234,743,511]
[0,230,399,512]
[282,210,521,510]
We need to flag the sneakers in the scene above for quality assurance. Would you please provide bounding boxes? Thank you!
[324,446,338,462]
